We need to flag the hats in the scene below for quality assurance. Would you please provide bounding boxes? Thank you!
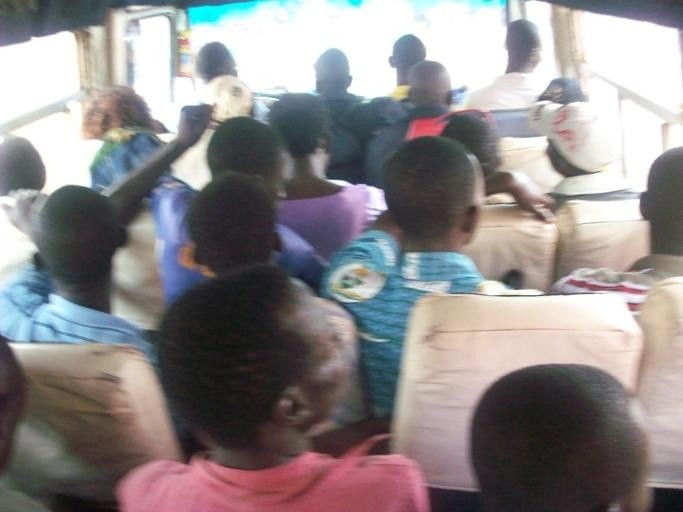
[193,76,252,123]
[541,79,584,103]
[532,99,616,171]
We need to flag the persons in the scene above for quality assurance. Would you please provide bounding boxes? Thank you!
[312,48,352,94]
[385,33,426,102]
[194,42,239,84]
[0,336,46,512]
[114,263,429,511]
[471,366,651,512]
[553,146,683,311]
[465,18,545,111]
[0,105,215,361]
[177,135,543,425]
[2,58,681,297]
